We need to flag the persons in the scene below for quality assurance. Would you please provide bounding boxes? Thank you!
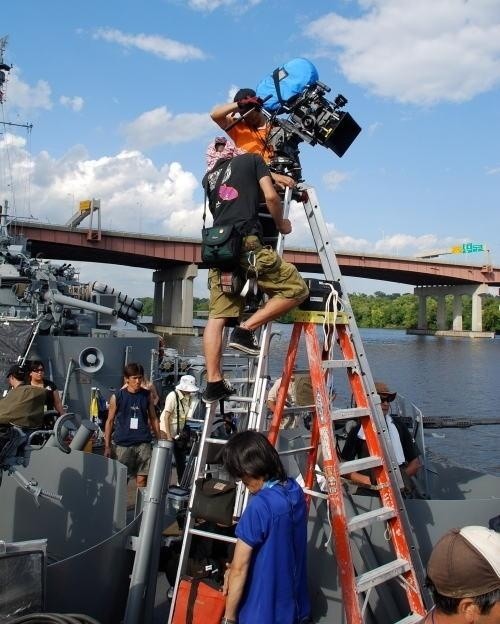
[0,365,55,471]
[23,360,66,442]
[393,524,500,624]
[266,361,311,430]
[209,88,278,312]
[105,362,159,489]
[202,136,310,403]
[160,374,213,486]
[220,431,312,624]
[121,375,159,407]
[339,381,423,497]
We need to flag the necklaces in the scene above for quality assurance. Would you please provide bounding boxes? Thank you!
[430,605,437,624]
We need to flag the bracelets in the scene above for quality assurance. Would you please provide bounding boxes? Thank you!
[221,617,236,624]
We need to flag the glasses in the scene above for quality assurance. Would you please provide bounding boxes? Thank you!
[30,366,44,373]
[379,397,393,403]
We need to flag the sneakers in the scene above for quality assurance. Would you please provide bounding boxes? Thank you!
[225,324,263,356]
[201,379,239,404]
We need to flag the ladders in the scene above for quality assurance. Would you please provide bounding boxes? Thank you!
[266,321,427,624]
[165,186,437,624]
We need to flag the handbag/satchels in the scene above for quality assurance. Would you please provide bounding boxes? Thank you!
[171,572,227,624]
[200,223,242,272]
[173,423,194,452]
[191,478,237,527]
[219,272,245,297]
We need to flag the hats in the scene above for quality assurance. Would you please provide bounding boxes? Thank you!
[5,364,25,377]
[425,524,500,601]
[175,374,202,392]
[375,383,398,401]
